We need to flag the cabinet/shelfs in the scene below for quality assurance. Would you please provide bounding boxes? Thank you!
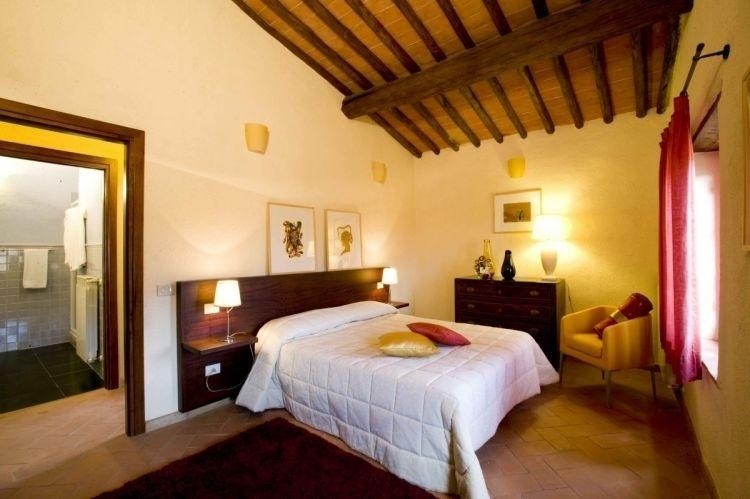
[453,274,567,372]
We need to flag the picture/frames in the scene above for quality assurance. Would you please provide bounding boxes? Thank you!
[474,239,497,280]
[492,189,541,234]
[266,202,317,275]
[323,208,363,271]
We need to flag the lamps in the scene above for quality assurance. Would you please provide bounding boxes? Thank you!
[531,213,563,282]
[214,279,241,342]
[382,267,398,304]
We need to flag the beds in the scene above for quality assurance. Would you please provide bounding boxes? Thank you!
[174,267,562,499]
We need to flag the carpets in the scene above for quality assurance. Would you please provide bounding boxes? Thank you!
[91,418,440,498]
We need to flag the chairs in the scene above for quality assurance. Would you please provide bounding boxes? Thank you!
[558,305,658,408]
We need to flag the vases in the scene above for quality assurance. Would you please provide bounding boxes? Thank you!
[500,250,516,280]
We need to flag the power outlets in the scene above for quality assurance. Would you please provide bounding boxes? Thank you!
[204,363,221,377]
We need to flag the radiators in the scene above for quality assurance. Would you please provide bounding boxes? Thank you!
[75,277,100,361]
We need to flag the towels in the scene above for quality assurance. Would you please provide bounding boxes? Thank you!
[61,207,87,272]
[22,249,47,289]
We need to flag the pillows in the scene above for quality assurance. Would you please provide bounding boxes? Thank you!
[379,331,438,359]
[593,292,651,339]
[408,322,474,347]
